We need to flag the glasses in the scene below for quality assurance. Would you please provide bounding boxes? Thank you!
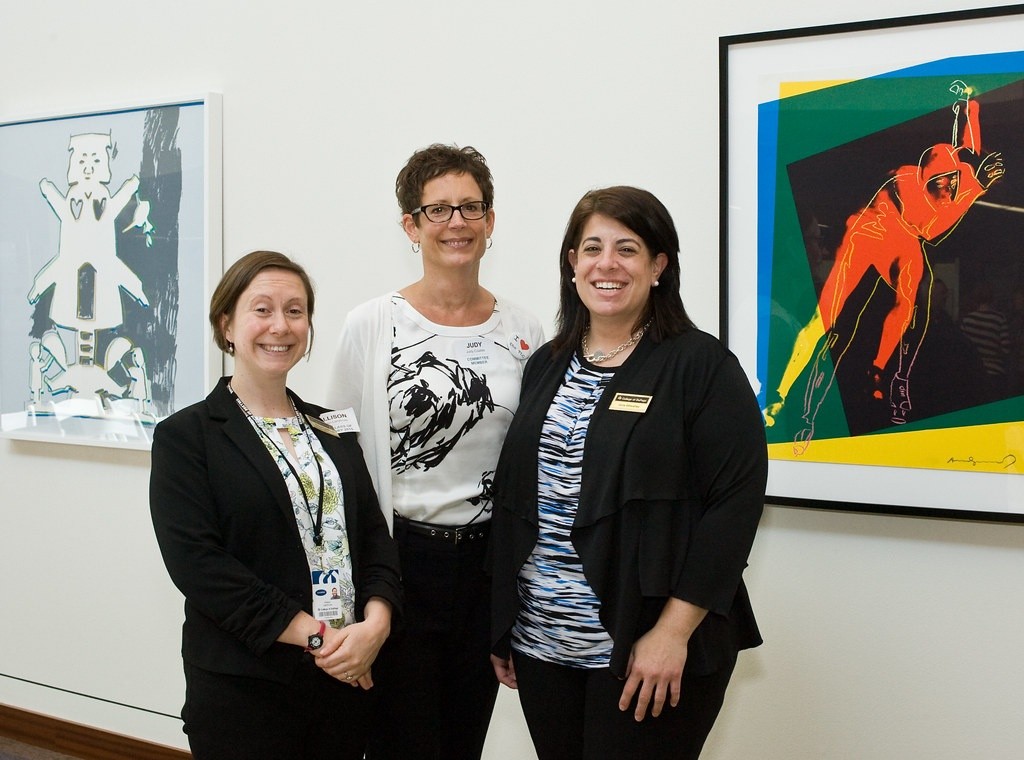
[411,201,489,223]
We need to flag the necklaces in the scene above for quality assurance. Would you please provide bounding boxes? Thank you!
[580,318,653,363]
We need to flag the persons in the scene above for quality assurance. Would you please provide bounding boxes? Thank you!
[330,587,339,599]
[801,206,1024,402]
[486,183,771,760]
[147,249,407,760]
[321,138,547,760]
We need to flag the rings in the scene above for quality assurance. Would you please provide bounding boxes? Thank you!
[344,672,353,681]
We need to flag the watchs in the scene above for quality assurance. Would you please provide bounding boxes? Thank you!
[304,619,326,653]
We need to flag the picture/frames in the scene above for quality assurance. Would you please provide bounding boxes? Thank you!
[0,93,225,453]
[717,4,1024,526]
